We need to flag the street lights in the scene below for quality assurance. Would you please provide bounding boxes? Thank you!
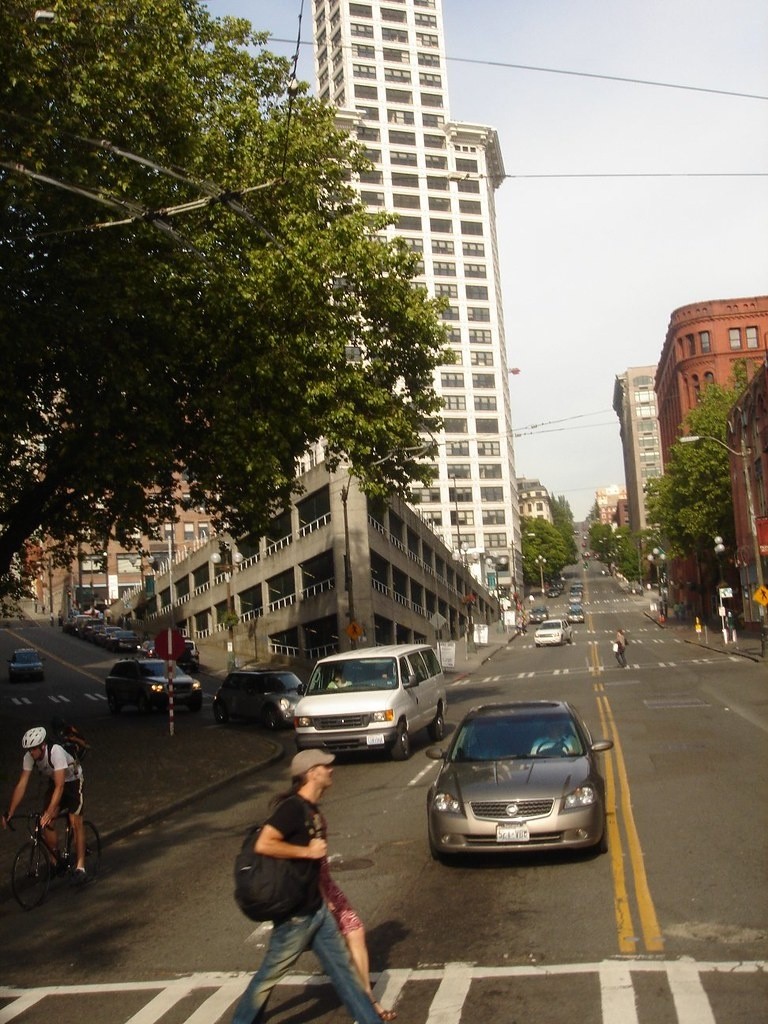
[510,532,537,609]
[482,558,509,630]
[209,538,244,675]
[713,536,736,645]
[125,551,157,641]
[535,553,548,602]
[679,435,768,660]
[646,547,667,619]
[452,541,481,655]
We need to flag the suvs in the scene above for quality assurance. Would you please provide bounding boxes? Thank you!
[534,619,573,646]
[105,655,203,716]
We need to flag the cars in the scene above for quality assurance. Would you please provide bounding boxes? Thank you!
[527,605,549,623]
[211,670,307,731]
[63,614,199,673]
[565,606,587,625]
[424,700,615,862]
[7,647,46,681]
[549,577,585,604]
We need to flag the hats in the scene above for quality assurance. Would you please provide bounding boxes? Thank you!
[290,748,336,776]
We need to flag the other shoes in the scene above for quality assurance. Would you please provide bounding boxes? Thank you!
[372,1001,397,1021]
[75,867,85,877]
[618,664,627,668]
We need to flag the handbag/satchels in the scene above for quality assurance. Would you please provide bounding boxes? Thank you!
[233,797,323,923]
[613,643,618,652]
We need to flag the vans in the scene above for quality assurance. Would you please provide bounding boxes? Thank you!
[293,642,447,761]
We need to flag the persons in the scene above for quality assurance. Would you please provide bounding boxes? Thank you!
[230,749,399,1024]
[530,720,573,754]
[673,602,685,621]
[50,611,54,626]
[382,674,407,683]
[41,605,45,613]
[68,608,83,618]
[615,630,627,667]
[327,668,352,689]
[105,607,112,625]
[515,616,527,635]
[117,613,132,630]
[58,610,64,626]
[725,608,744,640]
[0,727,86,876]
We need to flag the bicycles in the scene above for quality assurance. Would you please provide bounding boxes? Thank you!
[3,807,102,911]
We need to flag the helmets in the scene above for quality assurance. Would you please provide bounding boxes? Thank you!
[22,727,46,748]
